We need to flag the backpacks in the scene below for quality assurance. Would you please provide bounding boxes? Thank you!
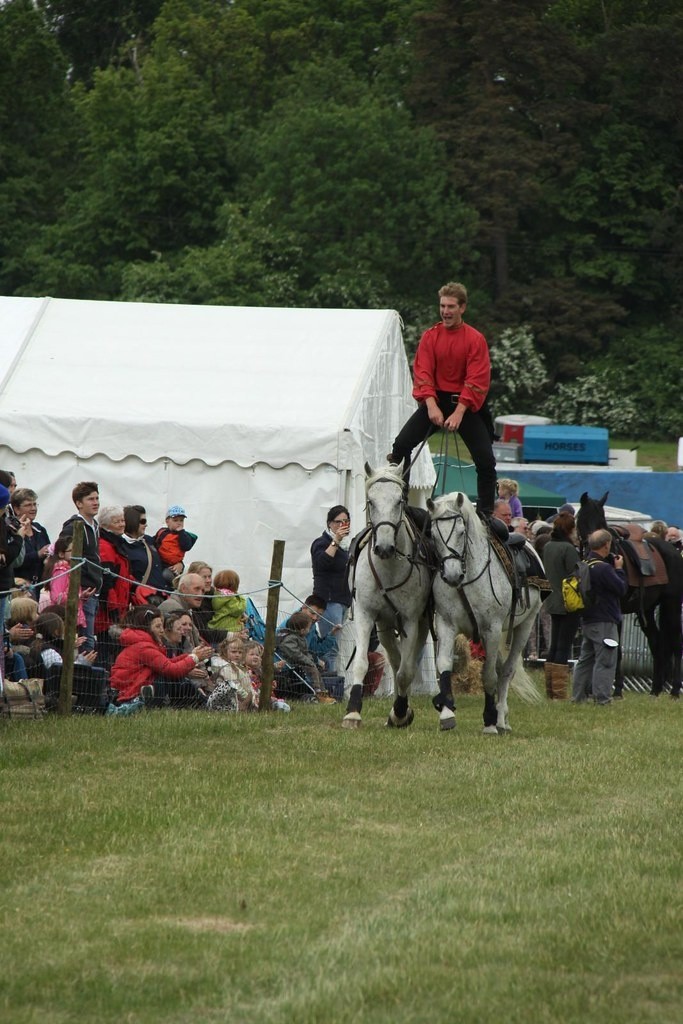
[562,559,604,613]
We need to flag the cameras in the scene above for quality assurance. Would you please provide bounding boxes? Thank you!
[604,553,619,565]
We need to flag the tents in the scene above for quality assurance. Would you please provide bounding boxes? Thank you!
[0,298,442,692]
[428,452,567,524]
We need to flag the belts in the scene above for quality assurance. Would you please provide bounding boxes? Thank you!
[436,390,460,404]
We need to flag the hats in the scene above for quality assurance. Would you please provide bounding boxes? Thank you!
[0,484,10,508]
[166,505,187,520]
[527,520,554,535]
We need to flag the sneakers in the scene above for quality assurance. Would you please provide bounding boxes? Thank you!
[315,689,337,705]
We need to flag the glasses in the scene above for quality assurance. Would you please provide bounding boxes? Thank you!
[333,520,342,524]
[23,503,39,508]
[143,609,155,618]
[140,519,146,524]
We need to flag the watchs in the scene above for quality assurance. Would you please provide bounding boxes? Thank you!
[331,541,339,547]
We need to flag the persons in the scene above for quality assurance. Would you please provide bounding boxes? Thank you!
[0,477,683,718]
[385,283,496,522]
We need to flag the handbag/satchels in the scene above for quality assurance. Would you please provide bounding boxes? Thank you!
[45,661,109,714]
[1,677,47,721]
[206,680,239,714]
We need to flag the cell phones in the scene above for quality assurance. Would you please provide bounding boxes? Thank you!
[342,519,350,534]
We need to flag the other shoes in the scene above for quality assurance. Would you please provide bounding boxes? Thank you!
[271,702,291,713]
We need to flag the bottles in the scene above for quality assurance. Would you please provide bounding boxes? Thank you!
[29,633,43,660]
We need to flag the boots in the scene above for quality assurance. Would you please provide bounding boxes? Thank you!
[392,443,412,505]
[544,662,574,702]
[476,476,495,526]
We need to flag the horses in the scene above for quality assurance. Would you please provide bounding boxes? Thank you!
[338,456,548,736]
[573,490,683,700]
